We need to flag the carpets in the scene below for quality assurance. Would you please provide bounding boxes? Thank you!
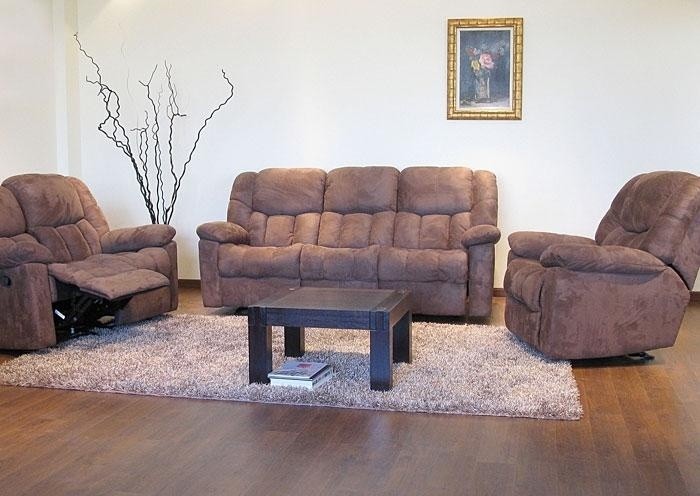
[1,313,584,421]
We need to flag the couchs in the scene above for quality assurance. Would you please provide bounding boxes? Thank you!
[499,171,696,361]
[2,173,179,355]
[196,166,501,321]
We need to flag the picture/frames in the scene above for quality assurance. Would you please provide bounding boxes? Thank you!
[447,17,524,121]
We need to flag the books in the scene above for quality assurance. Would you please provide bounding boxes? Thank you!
[270,368,336,389]
[267,360,329,378]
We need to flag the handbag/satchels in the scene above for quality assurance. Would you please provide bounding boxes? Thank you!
[51,294,98,342]
